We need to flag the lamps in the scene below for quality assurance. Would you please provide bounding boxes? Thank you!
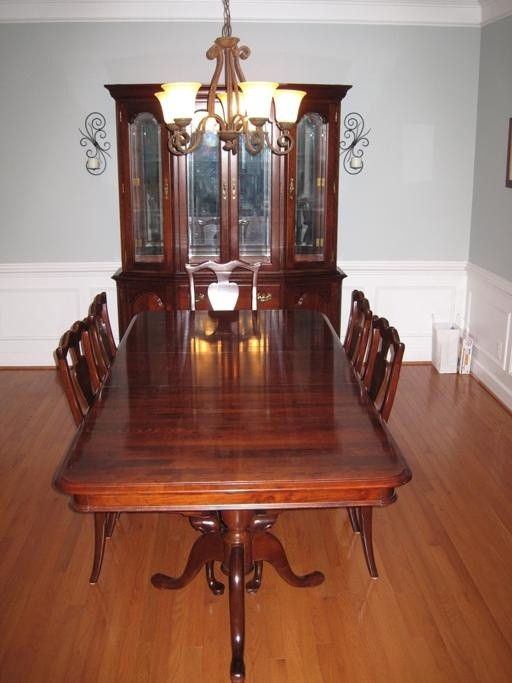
[154,0,307,156]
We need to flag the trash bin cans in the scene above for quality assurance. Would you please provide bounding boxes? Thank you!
[432,322,460,374]
